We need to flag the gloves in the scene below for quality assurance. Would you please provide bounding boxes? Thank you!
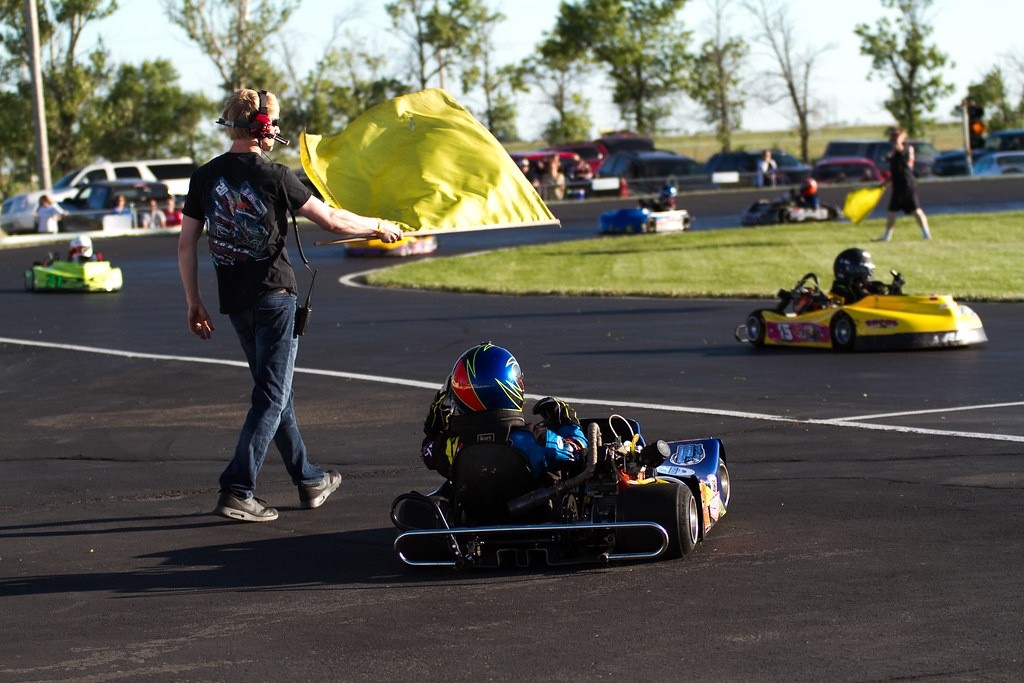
[532,396,582,434]
[422,390,452,437]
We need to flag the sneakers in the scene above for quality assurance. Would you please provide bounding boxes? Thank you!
[298,469,342,509]
[214,495,279,521]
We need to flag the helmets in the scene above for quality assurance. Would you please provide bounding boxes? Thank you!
[69,235,93,258]
[833,248,875,284]
[659,185,677,199]
[800,177,818,196]
[450,342,525,413]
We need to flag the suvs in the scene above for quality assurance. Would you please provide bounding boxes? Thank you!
[594,148,720,196]
[706,143,814,189]
[930,129,1024,176]
[0,155,202,236]
[507,131,658,201]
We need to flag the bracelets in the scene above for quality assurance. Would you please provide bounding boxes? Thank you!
[377,217,382,237]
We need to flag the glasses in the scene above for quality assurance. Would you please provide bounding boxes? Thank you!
[271,117,281,127]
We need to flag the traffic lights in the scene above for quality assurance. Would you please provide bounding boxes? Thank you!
[967,104,986,151]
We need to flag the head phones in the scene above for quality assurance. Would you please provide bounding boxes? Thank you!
[216,90,271,138]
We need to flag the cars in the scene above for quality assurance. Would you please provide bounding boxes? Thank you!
[811,156,891,184]
[971,150,1024,177]
[55,179,186,233]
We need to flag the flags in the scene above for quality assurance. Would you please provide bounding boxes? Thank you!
[299,87,557,237]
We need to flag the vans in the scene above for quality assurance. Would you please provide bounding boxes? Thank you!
[823,138,940,178]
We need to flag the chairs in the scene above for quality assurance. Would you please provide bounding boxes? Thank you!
[446,441,541,524]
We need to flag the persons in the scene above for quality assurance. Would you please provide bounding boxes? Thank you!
[760,150,777,186]
[178,89,403,520]
[112,194,136,229]
[69,234,98,263]
[570,153,593,179]
[870,128,931,241]
[536,159,546,179]
[550,153,566,178]
[162,195,182,227]
[797,178,819,212]
[542,159,565,200]
[638,185,677,212]
[33,195,68,233]
[828,248,905,305]
[420,341,588,471]
[517,157,540,187]
[141,199,167,229]
